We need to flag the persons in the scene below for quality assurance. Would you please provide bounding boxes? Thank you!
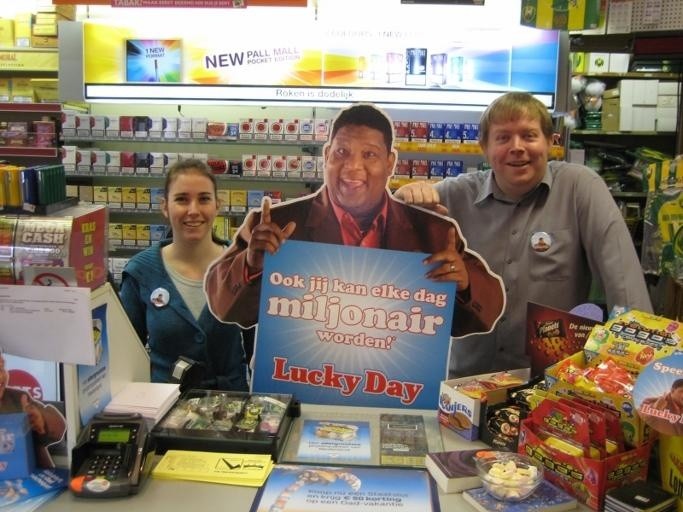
[637,379,682,436]
[399,88,656,379]
[118,158,252,393]
[203,104,508,339]
[0,355,66,469]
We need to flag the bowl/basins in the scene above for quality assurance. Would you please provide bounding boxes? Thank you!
[475,450,545,503]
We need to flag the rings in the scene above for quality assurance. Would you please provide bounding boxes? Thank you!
[450,262,456,272]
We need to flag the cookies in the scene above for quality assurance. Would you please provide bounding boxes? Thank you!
[449,412,472,431]
